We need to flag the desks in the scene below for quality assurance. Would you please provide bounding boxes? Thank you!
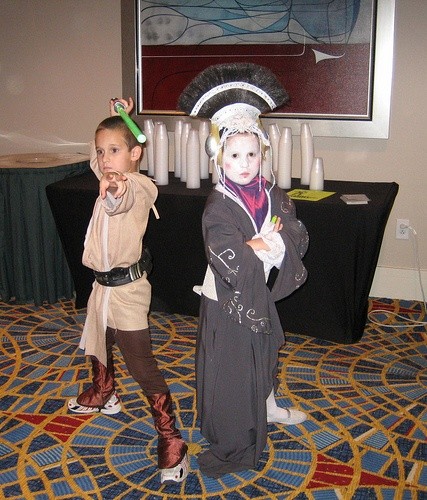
[0,154,90,307]
[46,170,400,345]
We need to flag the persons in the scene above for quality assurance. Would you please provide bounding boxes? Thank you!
[194,126,310,479]
[67,98,189,487]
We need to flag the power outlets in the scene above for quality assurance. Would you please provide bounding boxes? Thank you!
[396,219,410,240]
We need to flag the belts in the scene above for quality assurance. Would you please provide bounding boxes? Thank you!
[93,250,153,287]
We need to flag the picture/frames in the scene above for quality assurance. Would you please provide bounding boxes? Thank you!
[119,0,395,138]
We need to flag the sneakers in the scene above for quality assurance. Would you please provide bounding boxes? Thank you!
[161,451,188,485]
[68,392,121,414]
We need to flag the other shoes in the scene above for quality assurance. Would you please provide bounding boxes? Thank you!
[267,407,306,425]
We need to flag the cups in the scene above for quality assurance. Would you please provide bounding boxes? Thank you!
[143,118,324,191]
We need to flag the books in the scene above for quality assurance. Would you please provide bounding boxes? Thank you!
[339,194,370,205]
